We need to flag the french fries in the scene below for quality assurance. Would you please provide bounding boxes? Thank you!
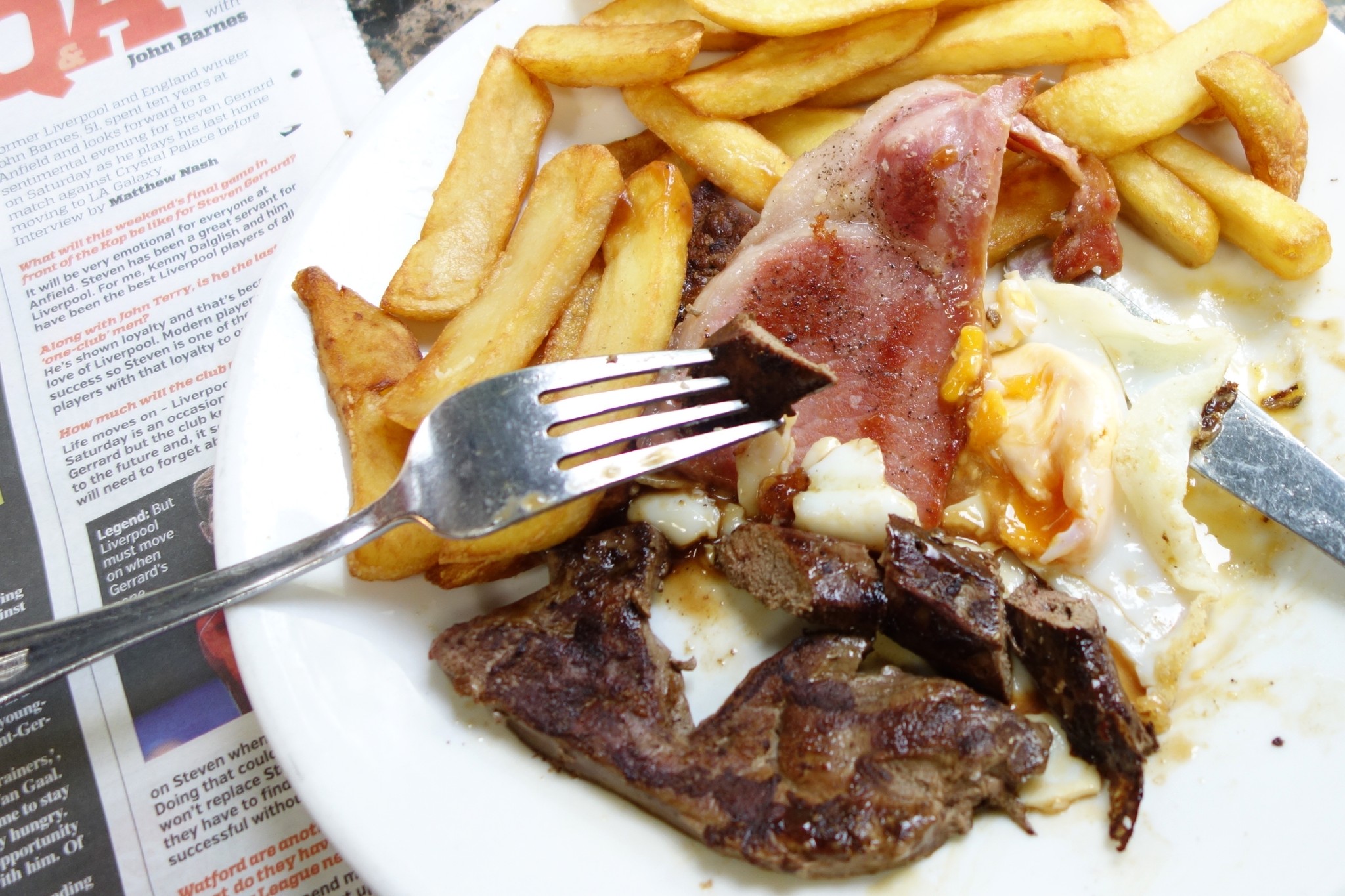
[289,1,1328,598]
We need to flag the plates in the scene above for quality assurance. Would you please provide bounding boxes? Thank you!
[203,0,1345,896]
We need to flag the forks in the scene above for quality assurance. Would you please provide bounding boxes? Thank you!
[0,350,782,703]
